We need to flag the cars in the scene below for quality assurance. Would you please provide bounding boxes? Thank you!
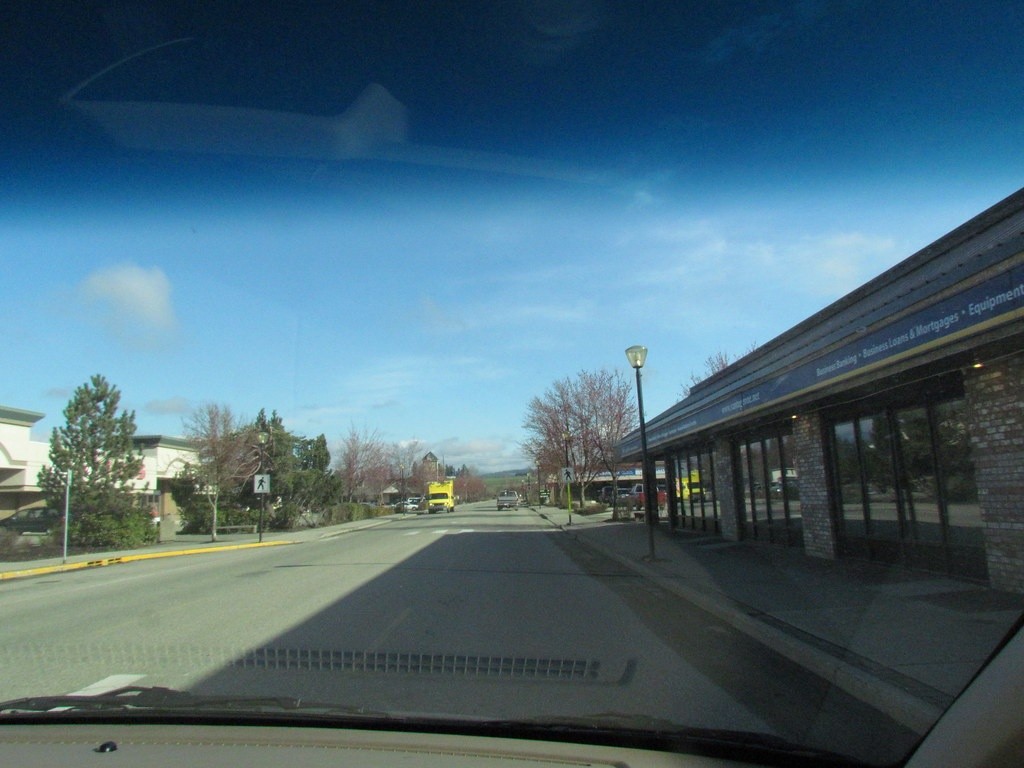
[596,482,667,511]
[743,477,802,499]
[0,506,66,535]
[391,497,422,511]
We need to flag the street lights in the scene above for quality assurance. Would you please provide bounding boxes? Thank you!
[623,346,658,562]
[256,430,270,543]
[536,460,542,509]
[561,431,574,525]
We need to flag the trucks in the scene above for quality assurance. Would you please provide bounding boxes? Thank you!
[428,480,454,514]
[680,477,706,504]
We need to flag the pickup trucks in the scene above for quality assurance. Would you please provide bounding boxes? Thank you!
[497,490,519,512]
